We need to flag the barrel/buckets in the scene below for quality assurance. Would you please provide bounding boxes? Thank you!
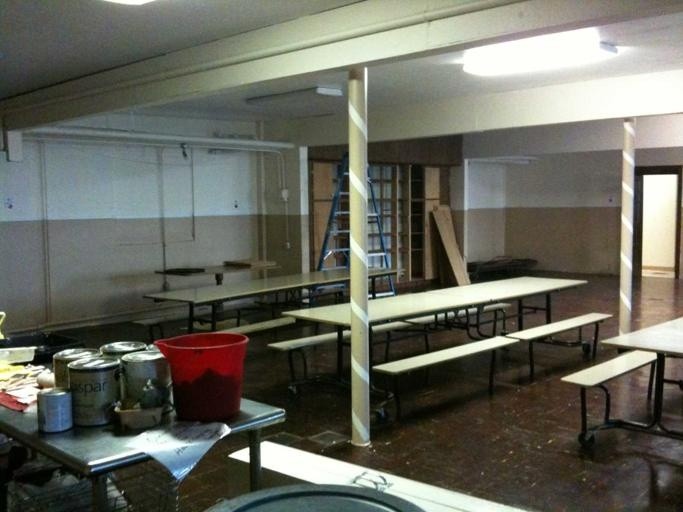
[153,332,248,421]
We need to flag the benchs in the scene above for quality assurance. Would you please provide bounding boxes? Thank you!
[133,262,682,460]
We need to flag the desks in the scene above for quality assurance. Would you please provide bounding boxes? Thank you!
[0,362,288,512]
[202,482,424,512]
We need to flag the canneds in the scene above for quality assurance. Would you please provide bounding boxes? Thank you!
[37,340,171,434]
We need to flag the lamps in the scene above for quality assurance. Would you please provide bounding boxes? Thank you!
[246,88,343,110]
[105,0,154,6]
[461,43,619,79]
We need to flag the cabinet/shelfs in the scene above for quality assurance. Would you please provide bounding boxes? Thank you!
[308,160,440,303]
